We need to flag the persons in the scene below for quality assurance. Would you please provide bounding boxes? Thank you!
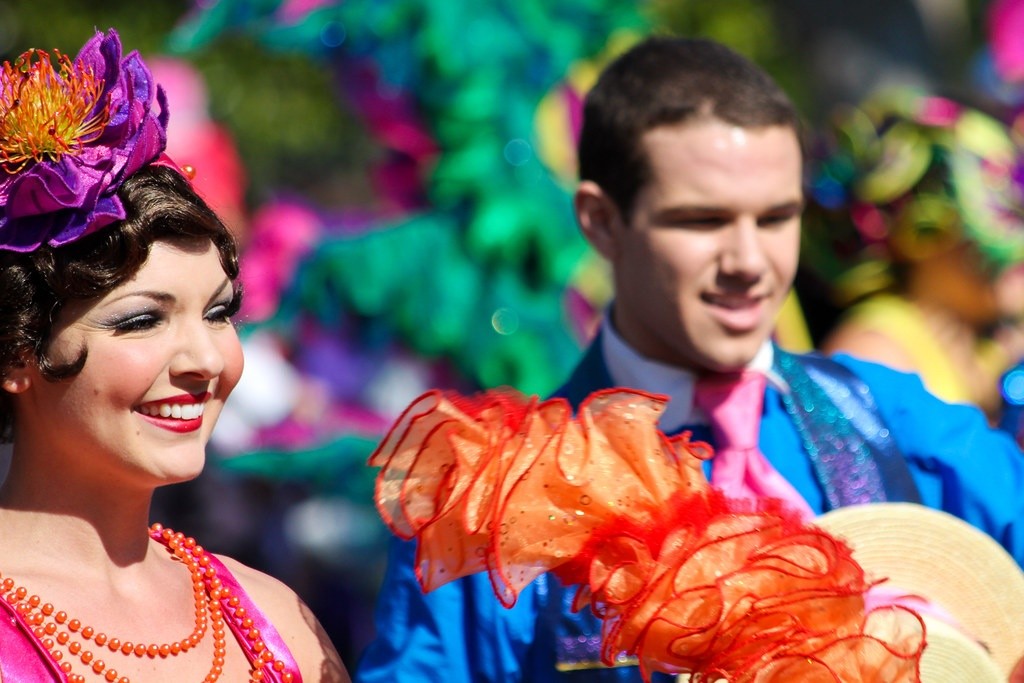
[0,162,355,683]
[359,34,1024,683]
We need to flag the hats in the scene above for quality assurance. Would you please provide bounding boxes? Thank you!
[673,501,1024,681]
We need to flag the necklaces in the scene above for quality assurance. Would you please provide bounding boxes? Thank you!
[0,521,294,683]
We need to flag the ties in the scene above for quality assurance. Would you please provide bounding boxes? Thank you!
[694,368,816,523]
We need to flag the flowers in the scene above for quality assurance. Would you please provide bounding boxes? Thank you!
[0,25,171,253]
